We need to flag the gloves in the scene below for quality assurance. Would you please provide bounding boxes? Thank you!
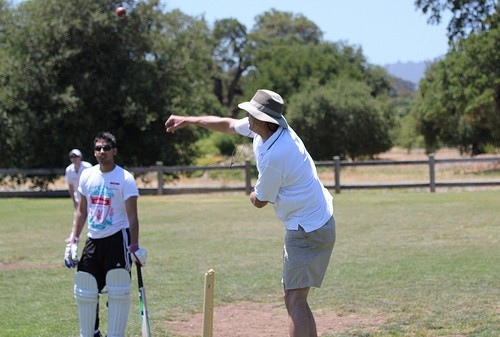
[130,244,148,266]
[64,237,80,268]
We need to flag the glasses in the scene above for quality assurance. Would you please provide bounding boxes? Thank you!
[70,156,80,158]
[94,145,113,151]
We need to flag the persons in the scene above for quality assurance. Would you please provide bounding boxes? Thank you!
[64,133,147,337]
[165,89,335,337]
[66,149,91,209]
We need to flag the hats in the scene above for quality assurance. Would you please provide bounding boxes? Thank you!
[69,149,82,158]
[237,89,288,129]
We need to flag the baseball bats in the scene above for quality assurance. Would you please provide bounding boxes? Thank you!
[135,261,152,337]
